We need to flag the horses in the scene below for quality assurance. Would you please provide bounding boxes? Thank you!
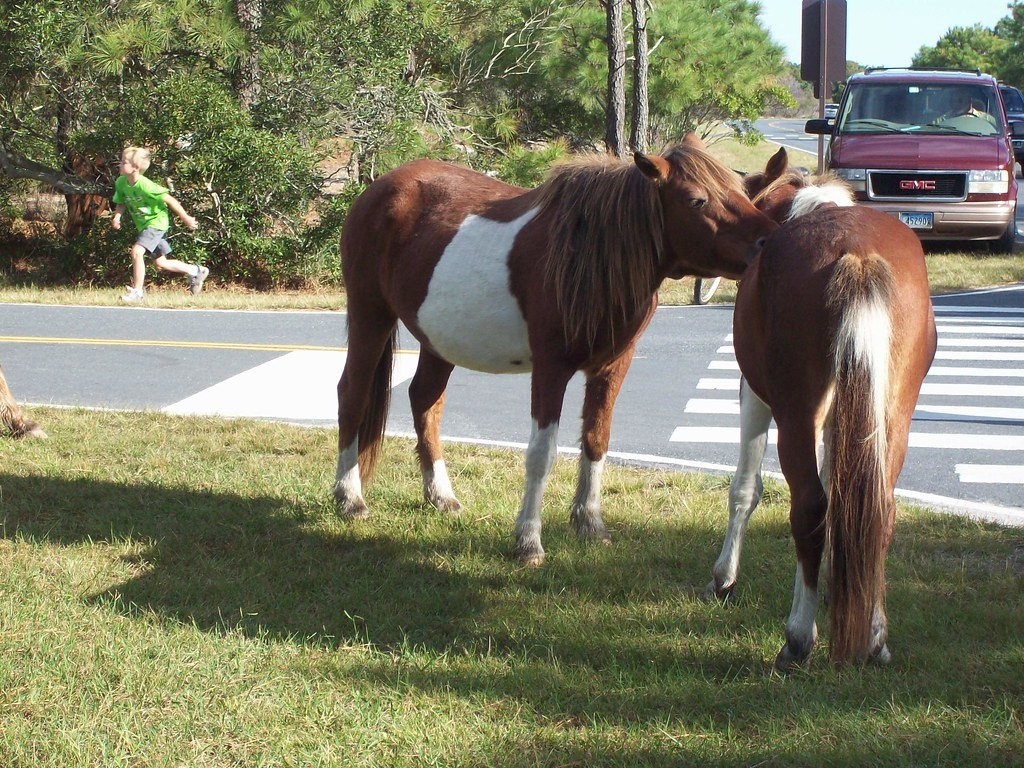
[331,131,779,571]
[707,146,938,683]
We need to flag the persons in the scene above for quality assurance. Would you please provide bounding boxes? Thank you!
[931,88,997,127]
[112,146,210,303]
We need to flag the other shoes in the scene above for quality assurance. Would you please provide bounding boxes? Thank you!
[123,287,144,303]
[190,265,209,295]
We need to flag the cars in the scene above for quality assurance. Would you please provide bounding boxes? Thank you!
[825,103,840,120]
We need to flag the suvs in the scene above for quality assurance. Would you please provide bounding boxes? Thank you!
[804,65,1023,254]
[998,78,1024,179]
